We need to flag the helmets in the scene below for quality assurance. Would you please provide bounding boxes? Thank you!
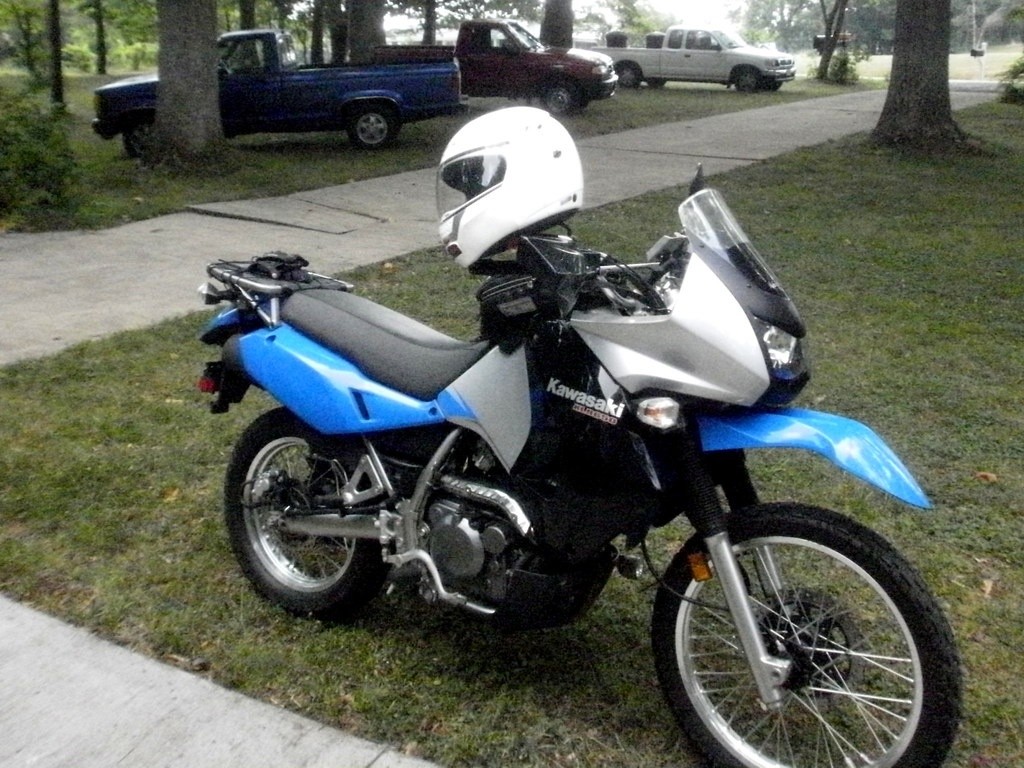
[434,105,587,269]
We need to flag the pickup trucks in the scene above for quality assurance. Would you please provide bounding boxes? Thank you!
[591,24,796,93]
[373,18,619,116]
[92,28,469,159]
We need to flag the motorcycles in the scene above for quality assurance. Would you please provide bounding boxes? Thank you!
[196,161,963,768]
